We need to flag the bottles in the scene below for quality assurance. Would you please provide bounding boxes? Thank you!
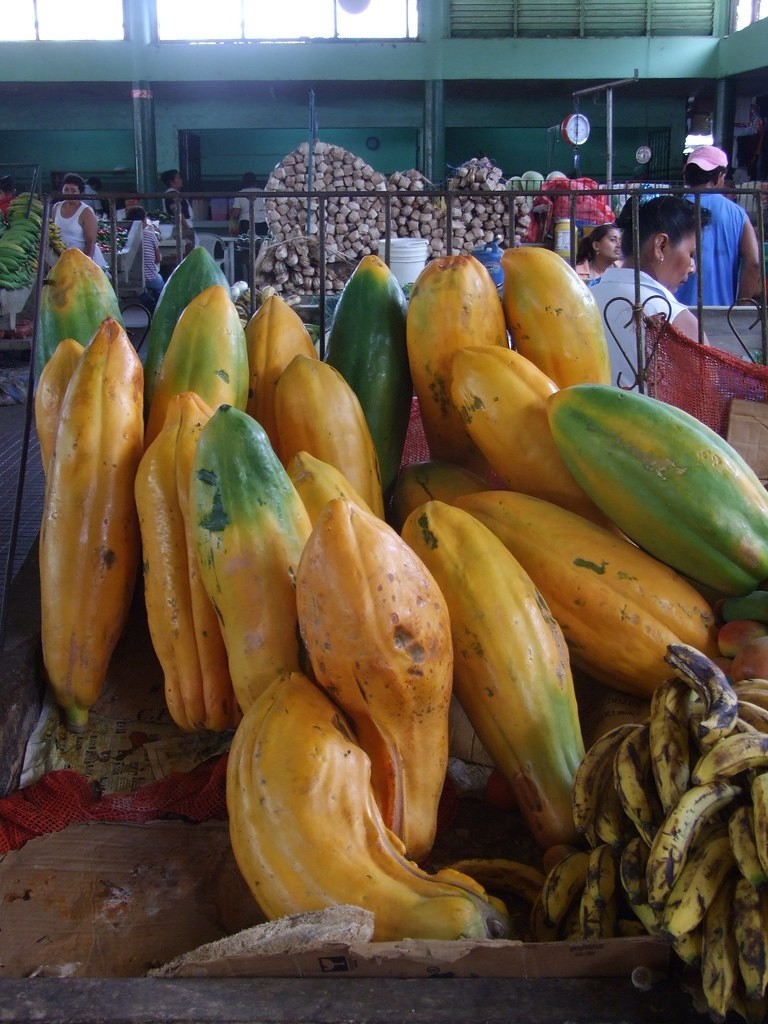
[555,218,577,257]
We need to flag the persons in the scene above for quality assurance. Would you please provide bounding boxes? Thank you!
[591,196,718,396]
[0,169,193,316]
[640,144,762,307]
[573,226,623,281]
[231,173,269,234]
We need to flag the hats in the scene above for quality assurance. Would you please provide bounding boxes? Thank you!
[684,146,729,172]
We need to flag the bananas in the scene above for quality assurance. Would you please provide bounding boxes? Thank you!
[428,642,768,1024]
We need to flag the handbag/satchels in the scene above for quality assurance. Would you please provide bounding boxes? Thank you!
[229,216,240,236]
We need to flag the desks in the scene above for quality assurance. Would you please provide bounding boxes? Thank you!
[158,237,237,294]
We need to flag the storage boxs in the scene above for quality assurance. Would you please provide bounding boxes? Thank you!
[97,220,143,272]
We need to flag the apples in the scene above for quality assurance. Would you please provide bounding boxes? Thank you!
[709,620,768,685]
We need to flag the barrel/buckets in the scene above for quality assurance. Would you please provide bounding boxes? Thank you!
[377,238,429,289]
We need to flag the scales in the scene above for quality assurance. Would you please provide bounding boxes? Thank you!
[562,94,591,170]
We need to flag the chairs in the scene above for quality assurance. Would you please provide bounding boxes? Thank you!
[196,235,230,283]
